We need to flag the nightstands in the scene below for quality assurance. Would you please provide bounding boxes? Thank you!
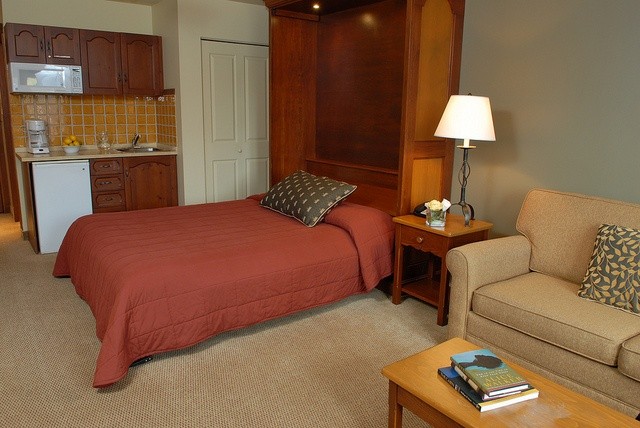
[392,214,494,327]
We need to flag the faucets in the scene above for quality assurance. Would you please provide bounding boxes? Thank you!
[132,133,141,148]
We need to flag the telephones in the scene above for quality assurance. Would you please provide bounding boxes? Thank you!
[413,200,427,218]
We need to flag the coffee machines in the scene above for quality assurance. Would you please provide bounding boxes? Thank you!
[24,118,51,154]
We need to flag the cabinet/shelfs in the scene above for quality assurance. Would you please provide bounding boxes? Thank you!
[4,22,79,65]
[79,29,163,97]
[89,156,178,214]
[201,37,269,204]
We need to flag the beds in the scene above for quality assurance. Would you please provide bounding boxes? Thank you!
[52,156,399,388]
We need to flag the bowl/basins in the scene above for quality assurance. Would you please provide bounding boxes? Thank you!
[62,145,80,155]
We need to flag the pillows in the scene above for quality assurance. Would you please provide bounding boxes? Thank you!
[578,223,640,315]
[260,170,357,227]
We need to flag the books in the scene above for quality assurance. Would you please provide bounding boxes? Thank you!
[450,349,529,397]
[438,367,540,413]
[451,362,522,401]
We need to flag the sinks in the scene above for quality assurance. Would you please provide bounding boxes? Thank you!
[116,146,162,153]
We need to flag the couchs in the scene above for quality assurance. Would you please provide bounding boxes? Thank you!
[445,188,639,419]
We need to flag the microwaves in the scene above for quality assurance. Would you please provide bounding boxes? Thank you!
[8,62,83,94]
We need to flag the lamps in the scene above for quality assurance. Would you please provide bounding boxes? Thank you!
[433,93,496,227]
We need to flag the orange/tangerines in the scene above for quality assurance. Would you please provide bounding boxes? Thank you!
[62,135,81,147]
